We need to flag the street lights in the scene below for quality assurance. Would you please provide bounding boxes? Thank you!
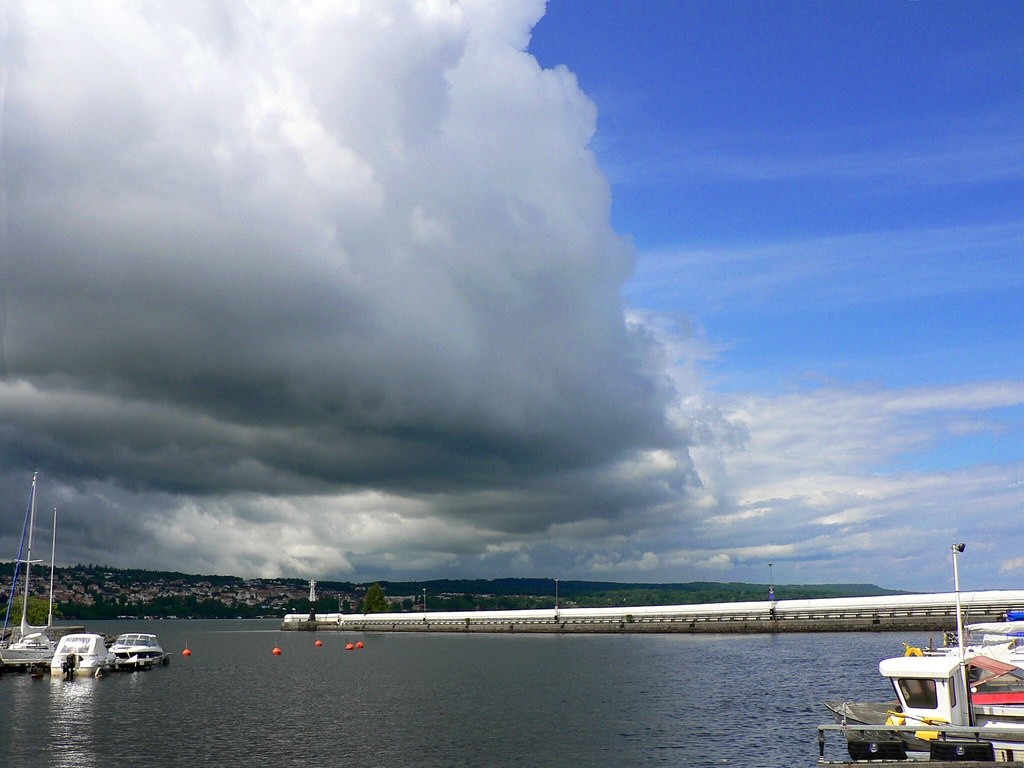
[423,589,427,618]
[767,562,775,610]
[554,578,560,613]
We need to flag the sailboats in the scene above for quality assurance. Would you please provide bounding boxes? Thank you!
[0,471,60,670]
[820,541,1024,763]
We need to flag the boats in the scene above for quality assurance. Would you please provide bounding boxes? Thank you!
[50,631,114,676]
[97,633,173,672]
